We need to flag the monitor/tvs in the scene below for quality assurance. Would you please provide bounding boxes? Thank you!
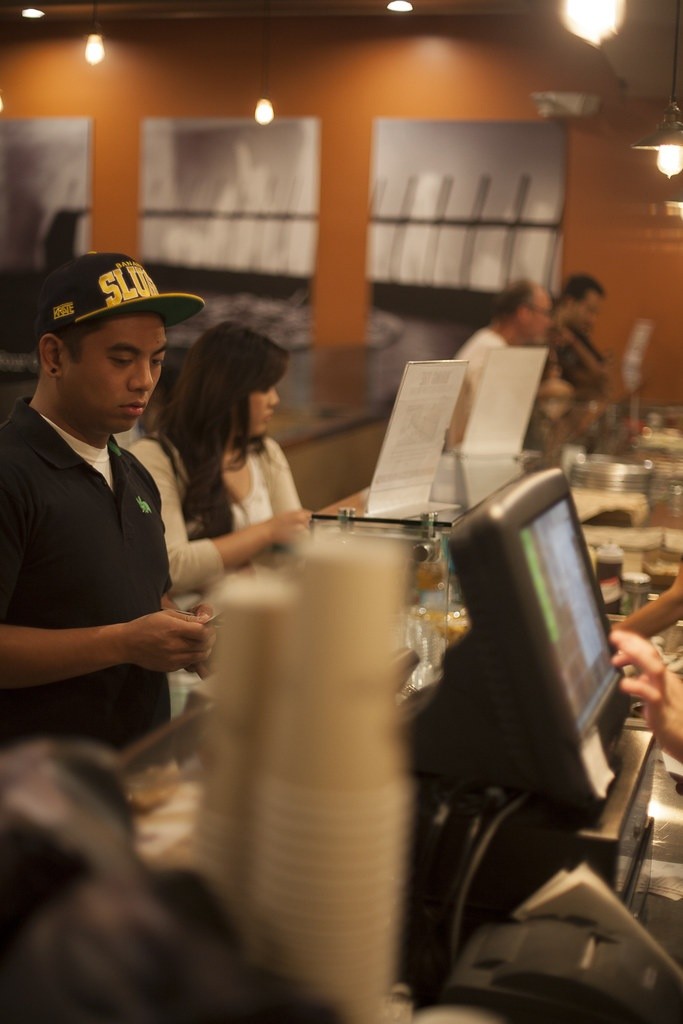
[447,468,633,806]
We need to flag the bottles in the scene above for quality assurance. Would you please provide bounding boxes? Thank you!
[596,537,653,619]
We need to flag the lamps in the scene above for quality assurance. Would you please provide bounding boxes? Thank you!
[630,0,683,179]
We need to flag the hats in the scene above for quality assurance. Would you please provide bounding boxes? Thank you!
[34,252,207,337]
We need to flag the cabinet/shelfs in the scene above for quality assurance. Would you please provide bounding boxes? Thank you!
[402,712,658,936]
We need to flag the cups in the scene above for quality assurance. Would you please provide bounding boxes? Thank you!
[198,534,406,1024]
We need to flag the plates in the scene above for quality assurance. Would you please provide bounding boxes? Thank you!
[571,449,682,522]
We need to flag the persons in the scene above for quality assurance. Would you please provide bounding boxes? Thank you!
[453,277,555,364]
[0,251,217,750]
[128,320,315,721]
[549,273,618,436]
[608,552,683,764]
